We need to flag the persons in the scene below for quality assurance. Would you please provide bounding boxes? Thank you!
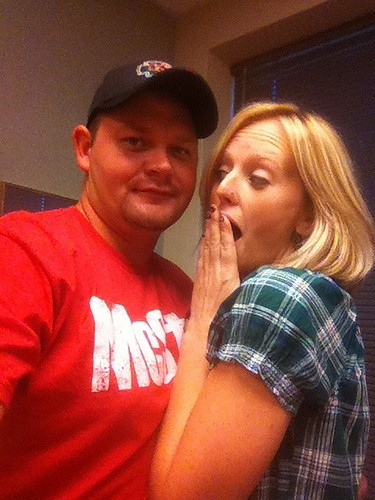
[0,60,232,500]
[149,98,373,498]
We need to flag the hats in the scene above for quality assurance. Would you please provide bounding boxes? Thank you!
[87,60,219,140]
